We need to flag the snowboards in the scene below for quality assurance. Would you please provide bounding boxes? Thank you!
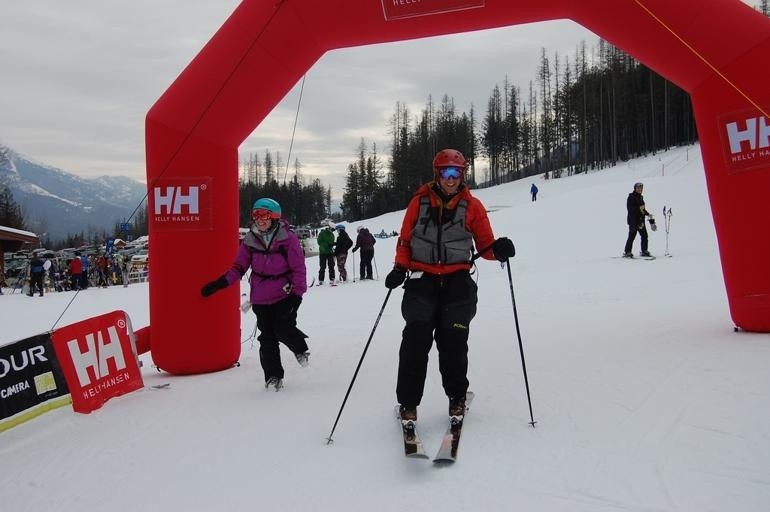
[21,260,51,293]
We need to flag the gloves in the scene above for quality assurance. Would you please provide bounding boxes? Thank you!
[281,295,302,321]
[352,247,358,252]
[649,219,658,231]
[491,236,516,262]
[385,265,406,288]
[202,276,228,297]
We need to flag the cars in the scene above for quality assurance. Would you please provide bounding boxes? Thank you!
[4,233,149,292]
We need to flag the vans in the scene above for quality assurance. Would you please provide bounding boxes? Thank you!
[239,227,250,240]
[294,217,337,240]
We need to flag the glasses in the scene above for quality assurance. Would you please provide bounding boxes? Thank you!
[435,166,467,180]
[251,213,272,222]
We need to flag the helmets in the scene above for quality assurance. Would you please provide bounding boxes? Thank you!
[433,149,467,184]
[335,224,345,233]
[634,182,644,191]
[357,225,364,232]
[329,222,336,231]
[251,197,282,220]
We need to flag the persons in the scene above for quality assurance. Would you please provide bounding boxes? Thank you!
[27,251,131,297]
[317,223,337,286]
[352,225,376,280]
[333,224,353,282]
[385,149,514,433]
[530,183,538,202]
[201,198,312,391]
[623,183,652,257]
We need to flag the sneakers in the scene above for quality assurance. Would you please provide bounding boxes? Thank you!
[297,348,313,369]
[449,396,468,422]
[623,251,634,257]
[640,250,654,257]
[399,404,419,427]
[265,376,284,393]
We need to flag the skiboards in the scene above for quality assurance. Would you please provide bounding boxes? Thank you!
[6,262,29,293]
[607,253,673,261]
[315,275,380,287]
[394,391,474,462]
[51,255,71,291]
